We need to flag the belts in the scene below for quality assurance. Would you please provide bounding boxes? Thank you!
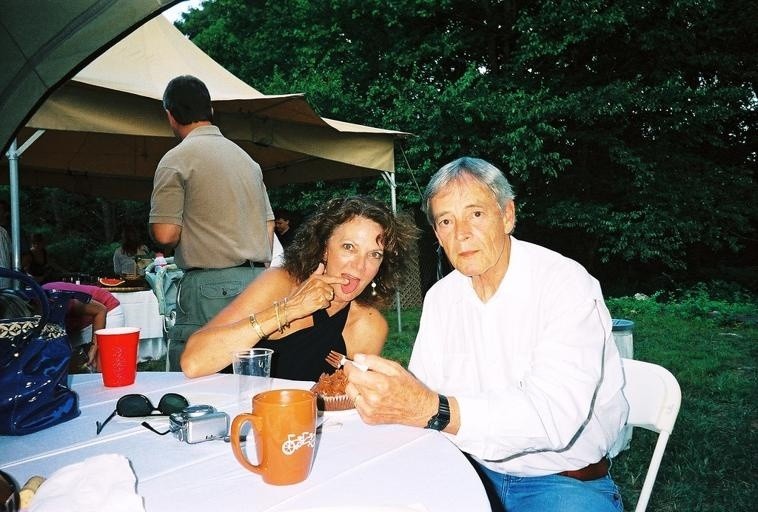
[559,458,614,484]
[185,259,266,274]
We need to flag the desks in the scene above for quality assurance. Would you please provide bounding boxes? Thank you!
[0,366,492,512]
[72,288,167,364]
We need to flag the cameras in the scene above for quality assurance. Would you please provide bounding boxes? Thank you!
[167,403,232,446]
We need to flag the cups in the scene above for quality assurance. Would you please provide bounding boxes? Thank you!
[232,348,274,404]
[228,388,317,487]
[91,325,140,389]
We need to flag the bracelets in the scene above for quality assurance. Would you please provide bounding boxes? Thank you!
[284,298,290,326]
[250,314,269,340]
[274,302,283,332]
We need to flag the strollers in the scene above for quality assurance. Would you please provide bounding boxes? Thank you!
[144,255,183,335]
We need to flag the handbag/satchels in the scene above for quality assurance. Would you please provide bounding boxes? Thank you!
[0,266,83,437]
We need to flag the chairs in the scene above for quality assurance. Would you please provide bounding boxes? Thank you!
[614,357,683,511]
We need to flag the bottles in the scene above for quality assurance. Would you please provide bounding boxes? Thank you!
[120,257,136,281]
[154,253,167,275]
[75,272,82,285]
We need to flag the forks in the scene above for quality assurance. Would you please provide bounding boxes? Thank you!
[325,348,370,372]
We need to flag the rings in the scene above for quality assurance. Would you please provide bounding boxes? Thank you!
[351,393,361,405]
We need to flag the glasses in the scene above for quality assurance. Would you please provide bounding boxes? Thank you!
[94,393,190,436]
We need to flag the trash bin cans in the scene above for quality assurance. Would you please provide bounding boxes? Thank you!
[611,318,635,359]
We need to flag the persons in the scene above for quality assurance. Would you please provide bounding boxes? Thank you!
[343,156,629,512]
[112,225,151,274]
[22,232,50,285]
[270,232,285,268]
[16,281,125,373]
[0,200,12,288]
[147,75,276,372]
[275,213,297,249]
[180,195,424,383]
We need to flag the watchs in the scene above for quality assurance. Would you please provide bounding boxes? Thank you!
[424,394,450,432]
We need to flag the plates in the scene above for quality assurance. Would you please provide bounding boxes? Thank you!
[100,278,126,286]
